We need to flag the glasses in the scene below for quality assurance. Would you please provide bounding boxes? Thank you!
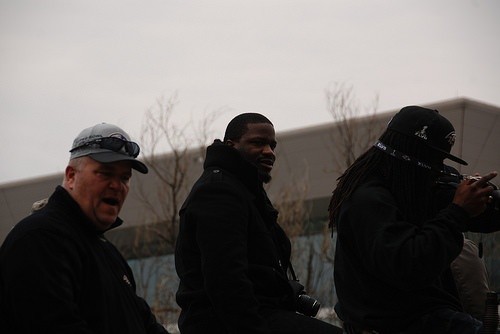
[69,137,140,157]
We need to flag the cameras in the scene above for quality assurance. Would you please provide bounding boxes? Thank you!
[283,279,320,317]
[450,176,500,216]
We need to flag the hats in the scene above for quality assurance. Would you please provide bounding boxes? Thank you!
[69,122,148,175]
[390,105,469,166]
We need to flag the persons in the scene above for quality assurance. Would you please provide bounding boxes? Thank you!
[0,122,168,334]
[329,106,500,334]
[175,113,343,334]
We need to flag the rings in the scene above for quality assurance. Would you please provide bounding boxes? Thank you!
[488,196,492,203]
